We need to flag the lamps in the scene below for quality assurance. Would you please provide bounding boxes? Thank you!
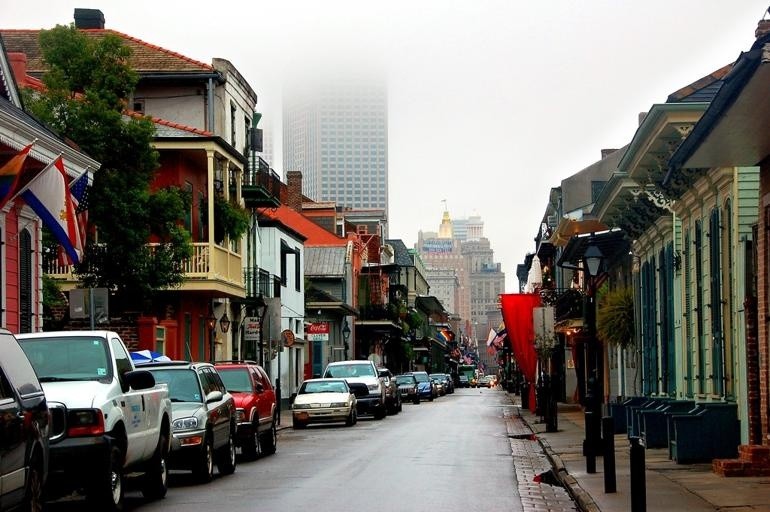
[220,298,230,332]
[206,296,217,330]
[342,314,350,339]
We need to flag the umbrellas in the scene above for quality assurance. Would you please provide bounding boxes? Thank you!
[130,348,171,365]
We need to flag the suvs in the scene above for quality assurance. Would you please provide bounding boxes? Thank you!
[0,326,59,511]
[208,356,280,460]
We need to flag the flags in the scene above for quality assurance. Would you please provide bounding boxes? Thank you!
[0,143,32,210]
[20,159,83,267]
[486,328,498,347]
[60,171,87,269]
[438,326,452,343]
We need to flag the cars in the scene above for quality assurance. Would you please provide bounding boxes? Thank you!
[289,376,358,430]
[326,358,499,422]
[121,356,240,483]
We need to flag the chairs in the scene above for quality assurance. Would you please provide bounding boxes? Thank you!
[609,397,741,465]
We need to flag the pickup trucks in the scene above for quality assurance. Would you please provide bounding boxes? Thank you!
[12,329,176,511]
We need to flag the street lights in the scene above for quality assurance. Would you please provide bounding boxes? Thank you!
[254,288,269,368]
[579,244,611,457]
[342,320,351,360]
